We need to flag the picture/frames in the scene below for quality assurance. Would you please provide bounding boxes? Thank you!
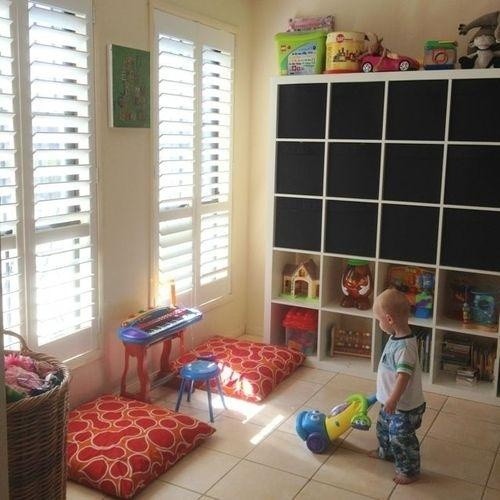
[105,43,151,128]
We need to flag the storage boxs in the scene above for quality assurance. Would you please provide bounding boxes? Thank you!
[274,30,328,75]
[281,306,319,354]
[424,39,457,69]
[470,288,497,325]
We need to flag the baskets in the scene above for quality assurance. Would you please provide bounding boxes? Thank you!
[2,330,72,500]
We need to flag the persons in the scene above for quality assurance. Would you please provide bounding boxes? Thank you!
[368,289,426,484]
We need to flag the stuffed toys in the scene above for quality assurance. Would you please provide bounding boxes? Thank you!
[458,9,500,69]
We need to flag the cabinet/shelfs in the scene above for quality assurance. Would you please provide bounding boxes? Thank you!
[264,69,500,408]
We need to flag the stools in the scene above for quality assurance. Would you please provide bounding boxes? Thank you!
[175,359,227,423]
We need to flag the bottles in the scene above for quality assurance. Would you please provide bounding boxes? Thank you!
[348,331,353,347]
[365,332,371,349]
[339,330,343,346]
[343,330,346,346]
[341,259,373,310]
[167,279,176,308]
[362,332,366,348]
[462,303,471,328]
[355,332,361,348]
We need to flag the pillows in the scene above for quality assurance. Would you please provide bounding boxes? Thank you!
[172,333,306,403]
[65,392,216,499]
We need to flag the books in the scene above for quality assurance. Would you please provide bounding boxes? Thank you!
[441,334,496,386]
[417,331,431,372]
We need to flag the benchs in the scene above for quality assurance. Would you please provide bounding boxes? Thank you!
[121,331,187,405]
